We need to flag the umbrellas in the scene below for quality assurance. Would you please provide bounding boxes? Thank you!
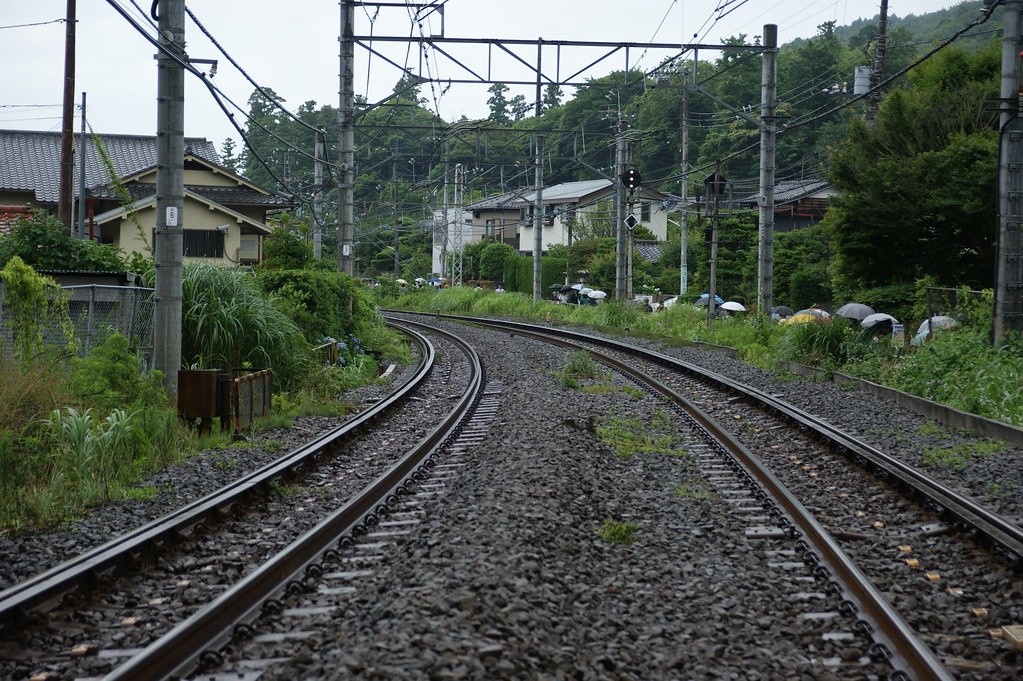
[720,302,746,311]
[560,286,572,292]
[396,277,447,284]
[549,284,562,289]
[578,288,594,295]
[571,284,585,290]
[784,303,899,328]
[693,294,724,305]
[774,306,795,315]
[588,291,607,298]
[917,313,957,335]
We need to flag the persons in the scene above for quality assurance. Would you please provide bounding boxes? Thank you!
[495,284,506,293]
[474,283,483,291]
[779,314,787,326]
[657,300,667,312]
[640,298,653,314]
[552,289,578,304]
[400,282,449,294]
[363,279,382,291]
[869,332,883,349]
[727,310,735,318]
[589,298,598,306]
[910,333,930,354]
[580,294,590,305]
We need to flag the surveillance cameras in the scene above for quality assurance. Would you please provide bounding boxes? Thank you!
[216,224,230,231]
